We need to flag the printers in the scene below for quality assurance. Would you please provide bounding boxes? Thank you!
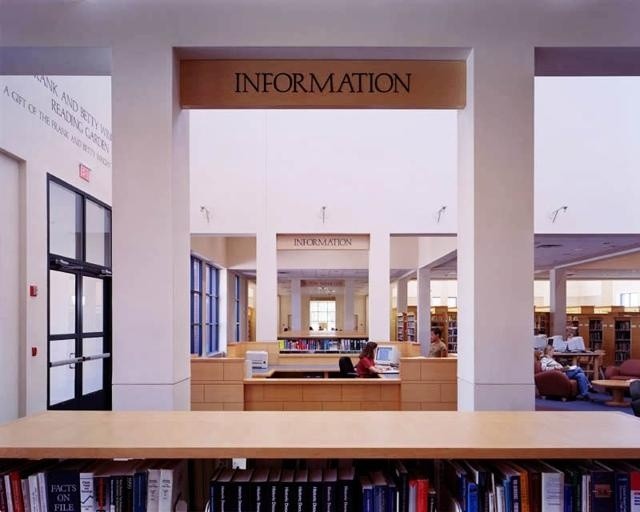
[245,349,268,369]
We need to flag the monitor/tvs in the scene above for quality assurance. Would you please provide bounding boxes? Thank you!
[373,345,400,366]
[534,332,585,353]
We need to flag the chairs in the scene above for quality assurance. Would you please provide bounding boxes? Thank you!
[534,362,580,402]
[605,359,640,396]
[629,379,640,417]
[339,357,360,377]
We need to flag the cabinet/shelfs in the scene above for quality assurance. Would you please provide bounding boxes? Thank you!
[431,306,448,351]
[535,312,640,371]
[448,308,457,352]
[0,411,640,512]
[278,332,368,353]
[395,313,415,341]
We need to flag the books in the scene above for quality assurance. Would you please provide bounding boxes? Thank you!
[614,320,631,362]
[277,339,367,351]
[1,459,639,512]
[589,321,602,350]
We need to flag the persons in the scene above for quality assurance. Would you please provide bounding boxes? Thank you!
[539,345,595,402]
[355,342,393,378]
[426,327,448,357]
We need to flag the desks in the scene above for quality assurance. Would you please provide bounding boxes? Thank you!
[591,380,631,407]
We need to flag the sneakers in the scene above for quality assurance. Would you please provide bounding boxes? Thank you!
[588,387,600,394]
[582,394,596,401]
